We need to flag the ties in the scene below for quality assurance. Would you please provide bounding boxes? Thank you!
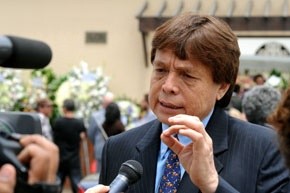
[156,136,182,193]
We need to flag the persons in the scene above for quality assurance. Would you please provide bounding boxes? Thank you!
[242,85,282,133]
[266,88,290,167]
[36,97,54,146]
[252,73,266,84]
[81,184,110,193]
[224,103,246,121]
[0,134,60,193]
[231,83,242,112]
[140,93,157,124]
[99,12,290,193]
[51,99,88,193]
[101,102,125,137]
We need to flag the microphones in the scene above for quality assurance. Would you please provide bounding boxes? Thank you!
[108,160,143,193]
[0,35,52,68]
